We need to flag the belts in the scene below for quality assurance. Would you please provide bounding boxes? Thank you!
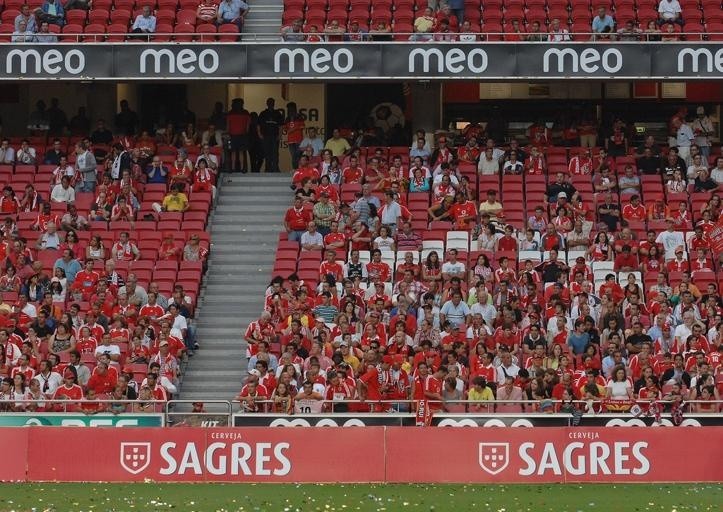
[383,223,395,226]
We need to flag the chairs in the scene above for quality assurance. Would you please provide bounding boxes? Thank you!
[2,2,248,42]
[0,145,222,416]
[283,0,723,42]
[271,146,720,414]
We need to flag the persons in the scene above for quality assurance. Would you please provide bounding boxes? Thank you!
[11,0,92,43]
[215,0,250,43]
[504,0,685,41]
[408,0,482,41]
[192,0,219,41]
[280,19,393,41]
[128,6,157,42]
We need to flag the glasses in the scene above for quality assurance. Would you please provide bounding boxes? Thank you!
[370,346,377,349]
[68,234,73,236]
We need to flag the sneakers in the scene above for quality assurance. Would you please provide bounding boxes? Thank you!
[290,185,296,190]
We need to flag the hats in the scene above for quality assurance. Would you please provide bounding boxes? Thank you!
[662,323,670,330]
[664,217,674,223]
[64,371,76,379]
[696,106,705,115]
[248,368,261,378]
[340,341,350,348]
[487,189,496,194]
[578,147,589,154]
[337,362,349,370]
[85,311,95,316]
[374,281,384,287]
[192,402,203,406]
[121,368,132,374]
[425,352,437,357]
[12,301,23,307]
[577,291,588,299]
[315,317,325,323]
[159,340,168,347]
[383,356,393,364]
[28,271,38,279]
[529,313,539,319]
[557,192,567,200]
[576,257,585,262]
[655,193,665,202]
[7,318,16,327]
[675,248,683,253]
[422,304,432,308]
[320,192,330,198]
[394,354,404,364]
[688,335,697,344]
[443,320,460,329]
[370,312,380,318]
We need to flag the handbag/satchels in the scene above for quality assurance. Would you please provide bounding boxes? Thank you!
[222,133,232,150]
